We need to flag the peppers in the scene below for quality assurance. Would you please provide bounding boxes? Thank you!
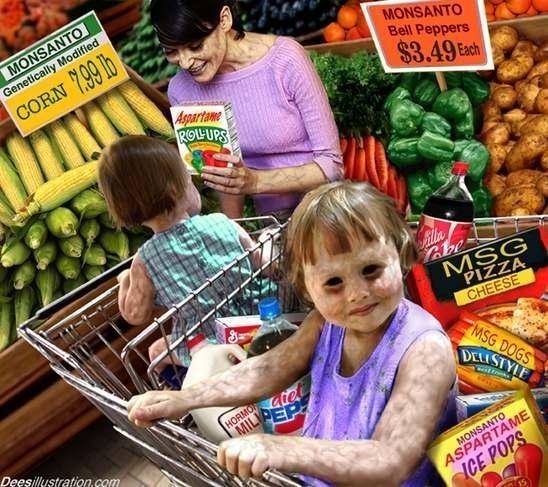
[381,72,493,218]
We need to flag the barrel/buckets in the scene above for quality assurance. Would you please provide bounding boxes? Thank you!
[180,335,266,446]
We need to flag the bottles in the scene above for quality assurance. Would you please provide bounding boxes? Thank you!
[413,161,475,265]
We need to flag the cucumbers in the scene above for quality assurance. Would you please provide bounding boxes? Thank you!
[117,1,181,85]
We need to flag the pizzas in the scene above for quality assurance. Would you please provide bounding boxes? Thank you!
[472,296,548,352]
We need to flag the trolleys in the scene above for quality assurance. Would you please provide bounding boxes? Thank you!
[13,214,548,486]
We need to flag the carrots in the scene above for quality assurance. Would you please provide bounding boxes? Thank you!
[340,133,408,214]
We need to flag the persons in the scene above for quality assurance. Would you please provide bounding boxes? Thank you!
[126,179,458,487]
[97,134,275,376]
[150,0,345,228]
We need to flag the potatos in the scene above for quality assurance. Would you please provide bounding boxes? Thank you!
[476,26,548,224]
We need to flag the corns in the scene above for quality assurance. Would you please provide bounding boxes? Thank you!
[0,80,176,354]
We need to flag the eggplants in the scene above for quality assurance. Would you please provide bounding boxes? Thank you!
[239,0,341,37]
